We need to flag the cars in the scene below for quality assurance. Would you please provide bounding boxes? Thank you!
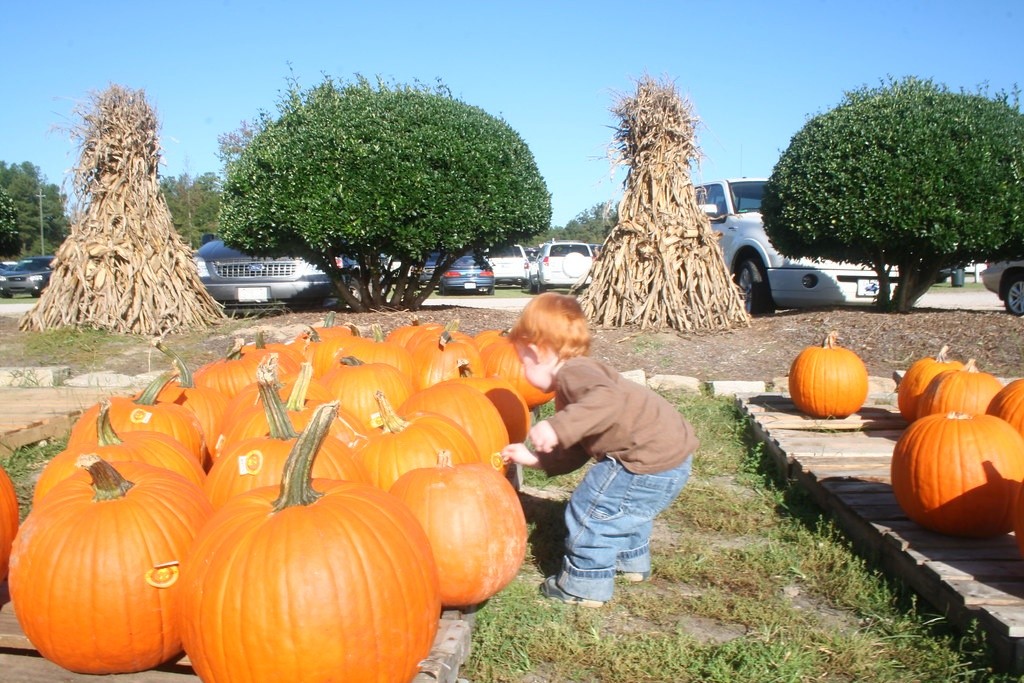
[980,255,1024,318]
[691,174,900,319]
[0,255,57,298]
[410,250,442,289]
[438,249,496,298]
[192,229,399,310]
[484,242,531,289]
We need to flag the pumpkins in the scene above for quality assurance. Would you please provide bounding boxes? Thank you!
[0,311,556,683]
[890,343,1024,565]
[789,332,868,419]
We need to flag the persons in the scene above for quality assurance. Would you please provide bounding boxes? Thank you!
[501,292,702,609]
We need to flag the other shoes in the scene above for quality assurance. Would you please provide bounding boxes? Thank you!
[540,575,608,608]
[613,570,649,581]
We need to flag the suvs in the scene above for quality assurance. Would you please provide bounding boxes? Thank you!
[523,237,604,295]
